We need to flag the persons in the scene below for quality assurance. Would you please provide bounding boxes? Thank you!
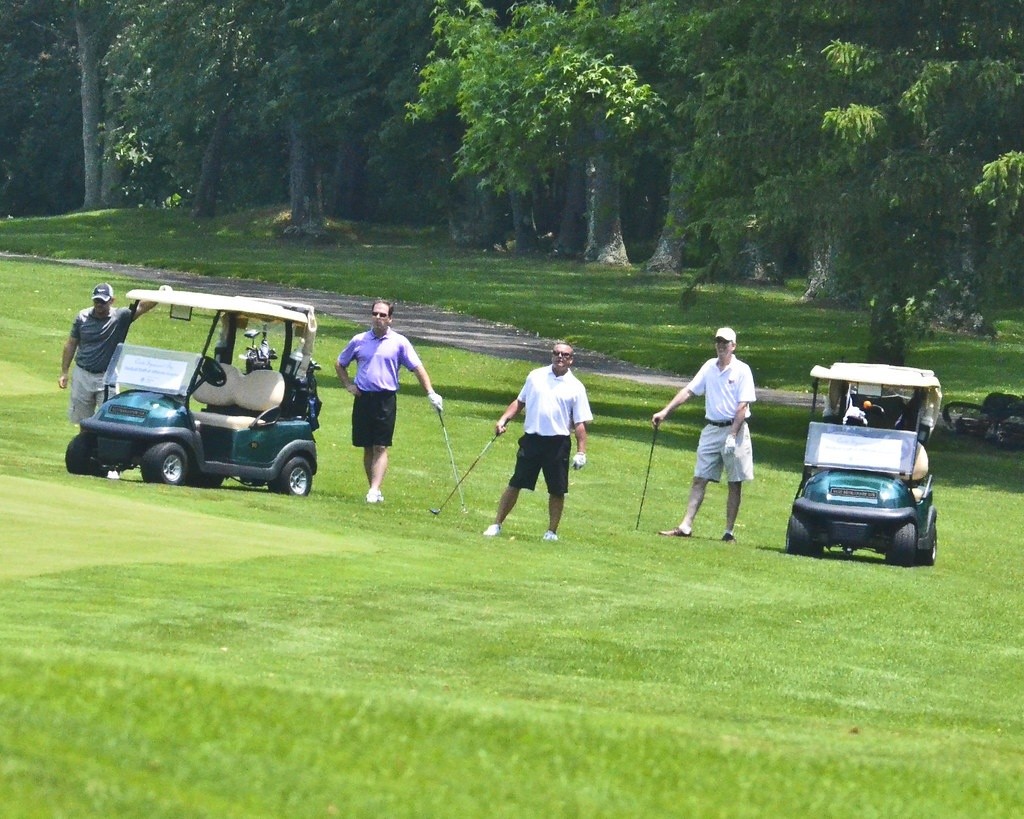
[652,327,756,541]
[335,300,443,502]
[483,344,593,540]
[59,283,173,433]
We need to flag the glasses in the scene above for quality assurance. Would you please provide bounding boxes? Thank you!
[371,312,387,317]
[716,339,731,344]
[553,352,570,356]
[93,297,111,305]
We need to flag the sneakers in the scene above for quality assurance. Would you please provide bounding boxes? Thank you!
[543,529,558,540]
[483,524,501,536]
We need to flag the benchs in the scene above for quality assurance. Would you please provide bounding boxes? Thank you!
[811,438,929,502]
[189,363,285,429]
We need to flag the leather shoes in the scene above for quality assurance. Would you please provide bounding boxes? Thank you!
[658,526,692,537]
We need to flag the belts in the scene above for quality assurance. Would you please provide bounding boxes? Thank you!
[708,420,735,428]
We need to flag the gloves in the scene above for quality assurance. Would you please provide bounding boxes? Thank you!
[723,434,735,456]
[159,285,173,291]
[572,451,586,470]
[427,389,444,411]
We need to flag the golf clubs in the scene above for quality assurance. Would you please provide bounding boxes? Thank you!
[437,409,468,516]
[428,417,512,516]
[308,361,323,377]
[239,323,278,363]
[635,418,661,530]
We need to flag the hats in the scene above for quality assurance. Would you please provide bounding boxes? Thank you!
[92,283,113,302]
[714,328,737,344]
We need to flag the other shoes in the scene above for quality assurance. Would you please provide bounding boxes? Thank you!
[106,470,121,479]
[366,489,384,503]
[722,533,735,540]
